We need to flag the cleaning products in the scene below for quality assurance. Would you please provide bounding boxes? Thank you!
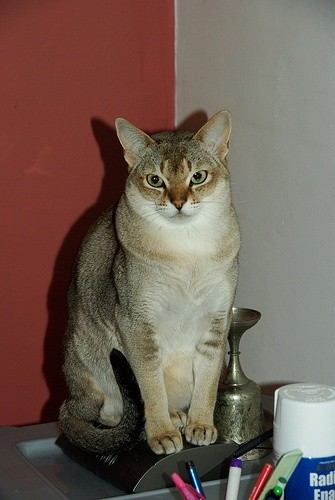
[272,383,335,500]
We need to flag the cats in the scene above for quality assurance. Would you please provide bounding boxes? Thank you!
[56,110,243,456]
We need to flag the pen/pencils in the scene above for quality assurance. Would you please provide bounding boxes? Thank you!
[171,449,306,499]
